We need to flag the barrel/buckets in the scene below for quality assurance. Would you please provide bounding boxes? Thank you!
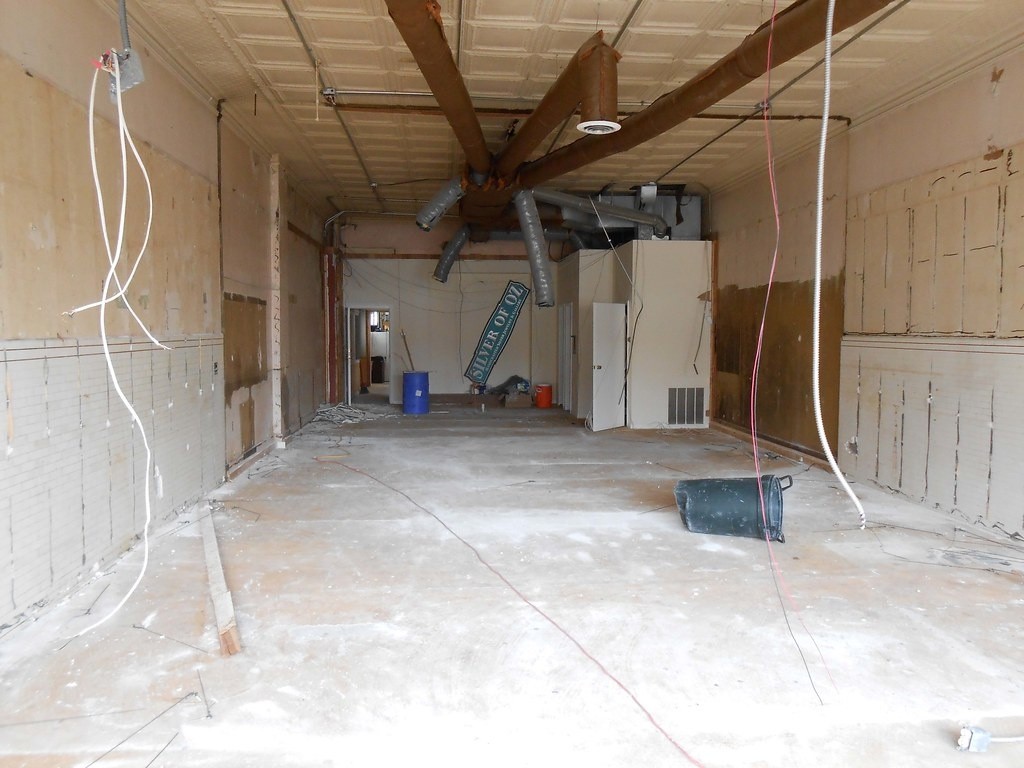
[674,475,793,544]
[402,372,430,414]
[536,383,553,409]
